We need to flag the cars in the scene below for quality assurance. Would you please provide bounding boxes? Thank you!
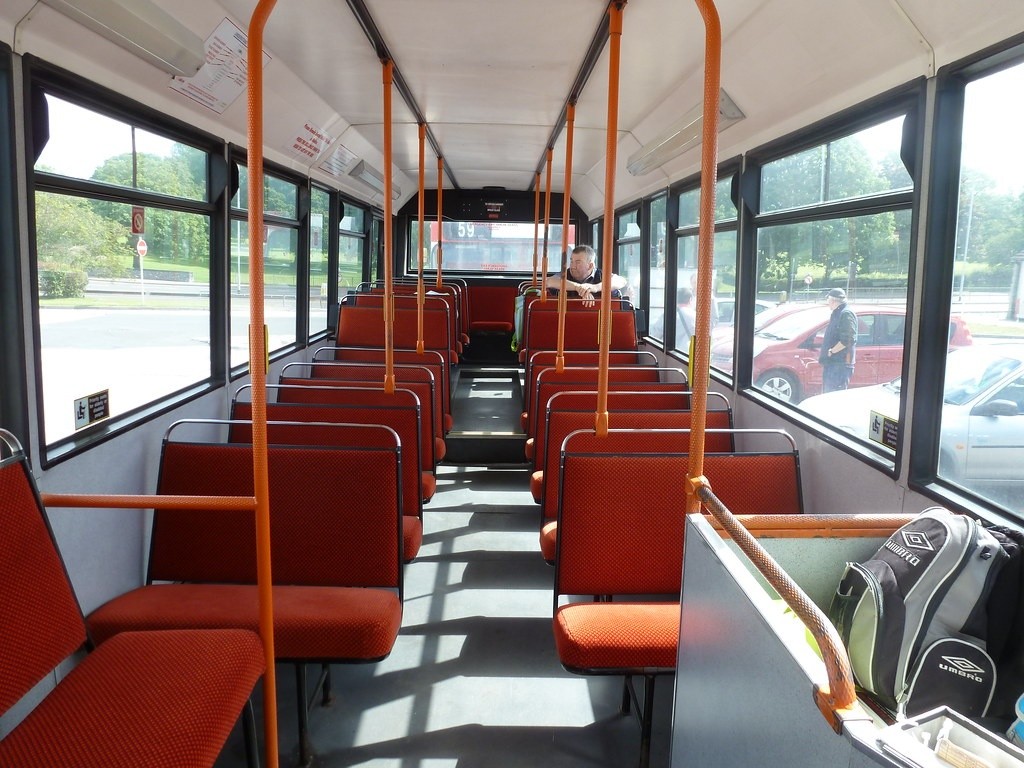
[701,296,975,406]
[798,341,1024,497]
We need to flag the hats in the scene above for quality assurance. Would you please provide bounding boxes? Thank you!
[829,288,845,298]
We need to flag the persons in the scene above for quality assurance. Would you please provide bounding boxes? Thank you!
[544,245,627,307]
[650,273,718,354]
[818,288,858,394]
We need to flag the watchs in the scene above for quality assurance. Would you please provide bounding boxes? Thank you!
[829,349,833,354]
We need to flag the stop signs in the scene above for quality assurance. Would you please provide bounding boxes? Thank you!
[137,240,148,257]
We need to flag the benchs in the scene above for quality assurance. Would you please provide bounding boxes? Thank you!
[277,362,436,504]
[540,391,735,567]
[333,294,453,435]
[311,347,446,466]
[84,418,404,768]
[520,299,639,434]
[228,384,423,565]
[551,429,805,768]
[0,429,266,768]
[531,367,691,505]
[525,351,661,463]
[354,277,550,366]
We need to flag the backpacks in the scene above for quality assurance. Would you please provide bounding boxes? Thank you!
[828,506,1024,733]
